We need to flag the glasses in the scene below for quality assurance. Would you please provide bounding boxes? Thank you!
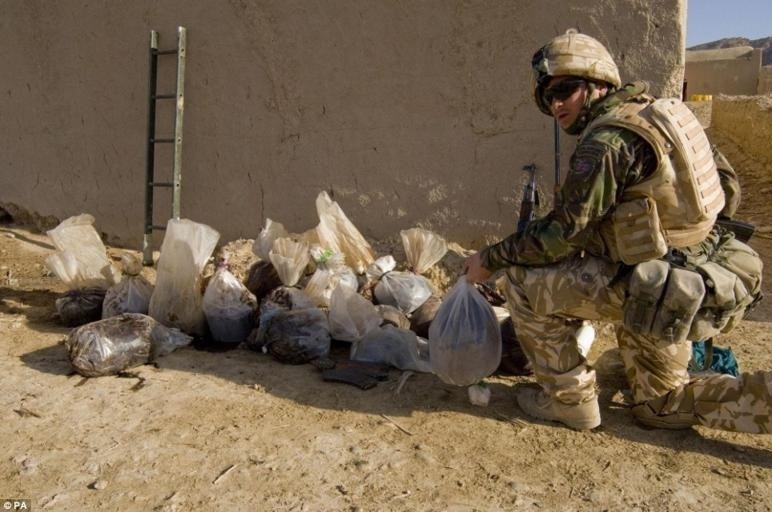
[543,76,587,108]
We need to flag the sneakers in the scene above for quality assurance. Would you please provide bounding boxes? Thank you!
[516,382,602,431]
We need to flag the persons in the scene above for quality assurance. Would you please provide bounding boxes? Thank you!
[461,27,772,435]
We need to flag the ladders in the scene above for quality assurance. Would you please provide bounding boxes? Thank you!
[142,27,186,252]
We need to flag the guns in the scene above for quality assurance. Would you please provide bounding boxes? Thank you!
[516,164,539,229]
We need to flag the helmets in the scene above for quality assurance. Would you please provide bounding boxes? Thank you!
[531,32,620,115]
